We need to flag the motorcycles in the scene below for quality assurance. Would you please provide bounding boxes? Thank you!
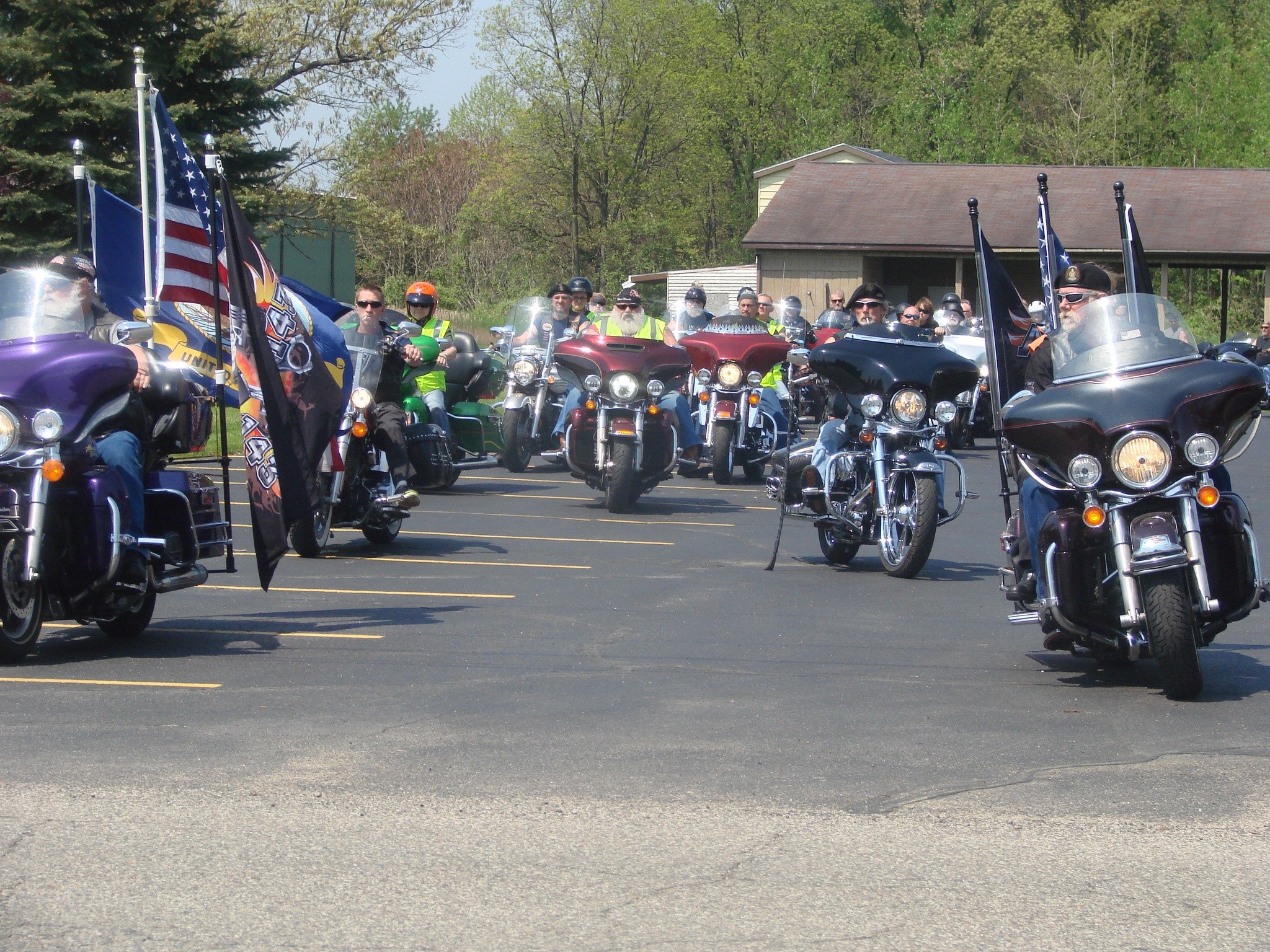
[667,300,994,486]
[0,262,240,666]
[765,320,982,579]
[968,290,1270,703]
[551,309,695,514]
[286,292,603,557]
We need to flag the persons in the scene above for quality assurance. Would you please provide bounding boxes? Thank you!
[402,282,460,464]
[0,250,151,622]
[1161,312,1190,346]
[1251,321,1270,407]
[998,264,1270,649]
[489,276,987,479]
[802,282,949,518]
[344,283,421,511]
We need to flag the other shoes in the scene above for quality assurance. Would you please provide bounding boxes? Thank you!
[398,489,420,509]
[539,448,568,467]
[938,507,949,520]
[800,465,828,515]
[677,459,713,478]
[120,550,147,583]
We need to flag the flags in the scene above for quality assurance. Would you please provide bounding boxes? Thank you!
[94,90,354,594]
[1119,201,1157,338]
[1037,196,1072,336]
[976,220,1048,409]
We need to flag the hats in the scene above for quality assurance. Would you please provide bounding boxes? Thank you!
[1054,263,1111,296]
[845,282,885,310]
[738,290,758,307]
[547,283,572,298]
[737,287,754,301]
[615,288,641,305]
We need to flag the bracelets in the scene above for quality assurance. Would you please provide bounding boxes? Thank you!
[441,351,450,360]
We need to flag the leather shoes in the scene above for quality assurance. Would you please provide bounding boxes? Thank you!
[1006,567,1037,601]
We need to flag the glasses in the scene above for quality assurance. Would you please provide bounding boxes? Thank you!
[573,296,588,302]
[903,314,920,320]
[615,304,639,310]
[832,299,843,304]
[357,300,384,308]
[1057,292,1098,304]
[409,302,431,308]
[854,301,883,308]
[759,302,772,306]
[1260,326,1270,329]
[918,308,929,314]
[590,301,606,306]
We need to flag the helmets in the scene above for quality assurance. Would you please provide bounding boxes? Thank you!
[684,287,707,310]
[784,296,802,316]
[943,292,961,305]
[405,282,437,318]
[943,303,965,319]
[1028,300,1045,313]
[568,277,593,297]
[896,302,910,322]
